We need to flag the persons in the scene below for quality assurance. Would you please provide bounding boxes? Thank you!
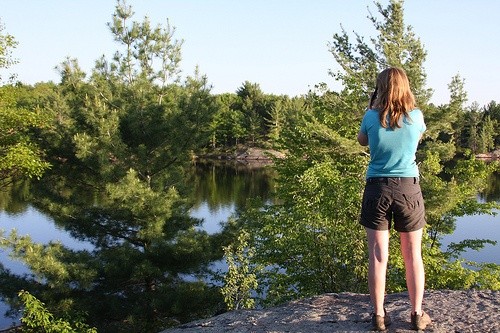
[358,67,433,331]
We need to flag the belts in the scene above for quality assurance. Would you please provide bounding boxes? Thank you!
[366,177,420,184]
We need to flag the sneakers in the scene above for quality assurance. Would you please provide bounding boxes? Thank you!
[370,308,391,330]
[411,310,431,330]
